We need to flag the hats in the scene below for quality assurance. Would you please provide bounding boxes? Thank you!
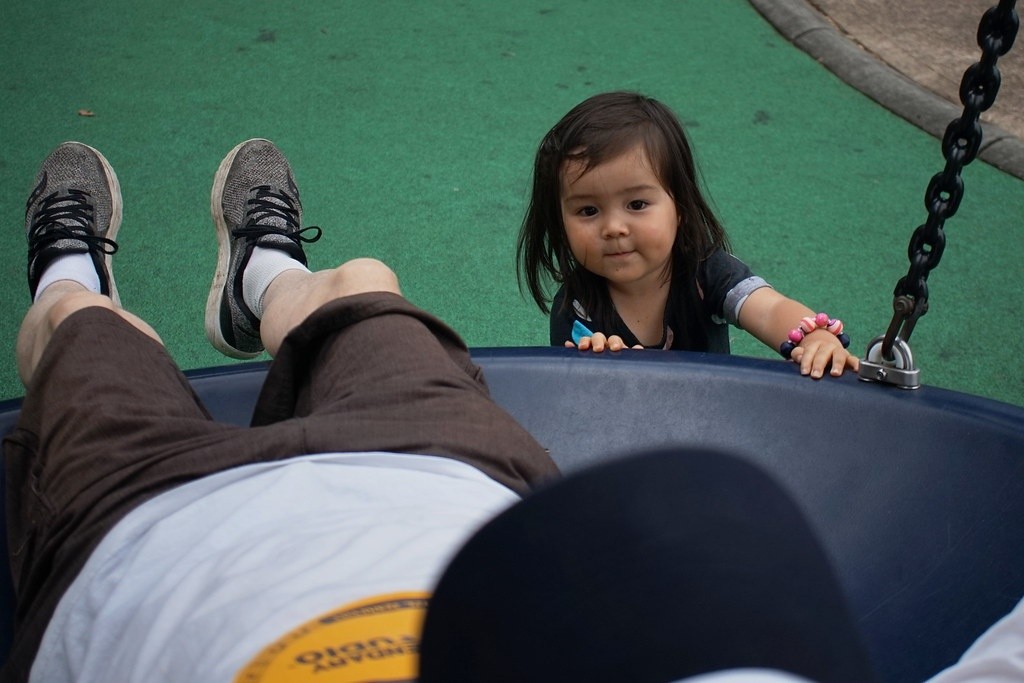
[418,445,875,683]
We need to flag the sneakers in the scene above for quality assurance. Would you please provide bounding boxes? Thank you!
[24,141,123,309]
[204,138,322,357]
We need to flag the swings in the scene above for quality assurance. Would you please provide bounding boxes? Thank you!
[0,1,1023,682]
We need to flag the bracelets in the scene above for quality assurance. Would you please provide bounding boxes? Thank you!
[779,312,851,360]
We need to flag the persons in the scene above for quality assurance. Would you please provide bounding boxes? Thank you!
[13,139,874,683]
[517,91,858,380]
